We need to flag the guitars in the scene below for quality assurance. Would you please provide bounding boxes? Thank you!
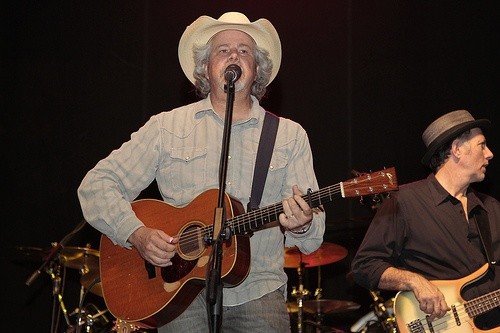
[99,165,399,329]
[394,263,500,333]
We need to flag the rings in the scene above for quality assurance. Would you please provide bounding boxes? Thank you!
[287,214,293,219]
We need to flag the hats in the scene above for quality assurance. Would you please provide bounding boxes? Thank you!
[421,110,492,163]
[177,12,282,95]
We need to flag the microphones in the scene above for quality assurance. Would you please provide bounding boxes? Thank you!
[224,64,242,82]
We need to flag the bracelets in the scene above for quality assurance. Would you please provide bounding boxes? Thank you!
[291,222,312,234]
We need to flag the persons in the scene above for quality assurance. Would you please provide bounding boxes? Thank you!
[78,12,326,333]
[348,109,500,333]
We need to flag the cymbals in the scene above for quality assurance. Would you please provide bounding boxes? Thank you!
[287,300,360,317]
[284,238,348,268]
[55,246,103,296]
